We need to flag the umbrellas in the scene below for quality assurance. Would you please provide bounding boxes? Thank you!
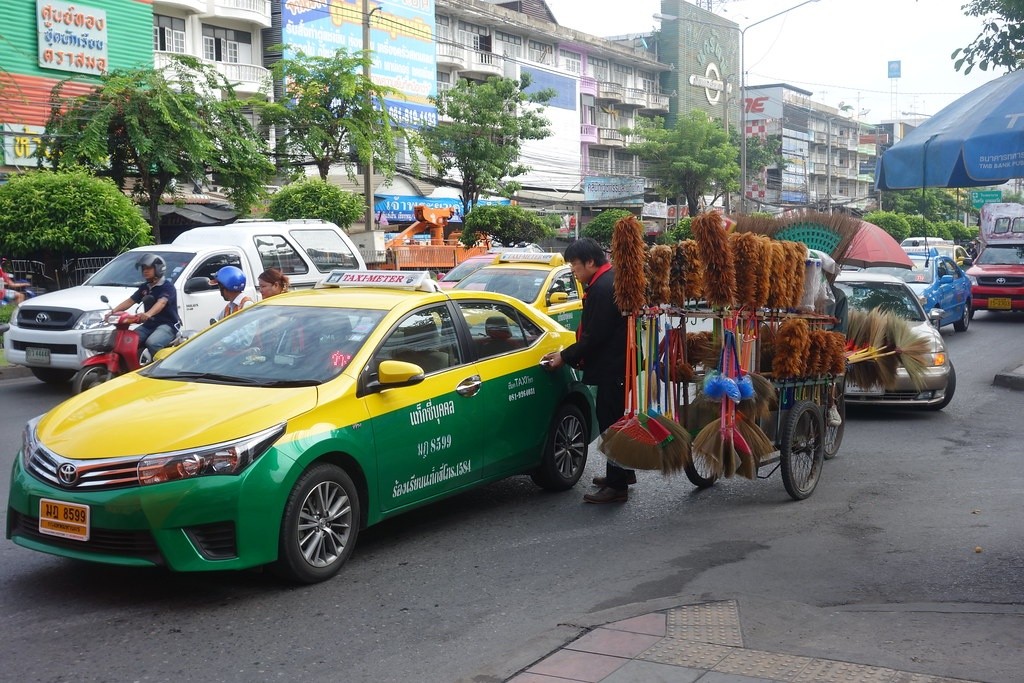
[874,69,1024,191]
[837,219,917,271]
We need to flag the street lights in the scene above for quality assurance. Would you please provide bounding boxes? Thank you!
[653,13,743,215]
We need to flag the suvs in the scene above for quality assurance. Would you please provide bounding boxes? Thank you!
[4,217,363,383]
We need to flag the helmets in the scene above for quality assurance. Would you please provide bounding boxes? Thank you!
[210,266,246,293]
[912,239,919,246]
[134,252,166,279]
[969,242,975,247]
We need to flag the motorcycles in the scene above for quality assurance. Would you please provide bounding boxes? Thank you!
[71,296,189,396]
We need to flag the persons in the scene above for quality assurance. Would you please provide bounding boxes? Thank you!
[105,255,180,363]
[966,242,977,262]
[821,285,848,426]
[0,258,31,305]
[545,238,637,504]
[169,266,290,365]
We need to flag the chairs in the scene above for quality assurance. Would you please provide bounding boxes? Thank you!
[392,316,450,373]
[304,314,360,374]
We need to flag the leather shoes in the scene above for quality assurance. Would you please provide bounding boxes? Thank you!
[592,476,636,485]
[583,486,629,504]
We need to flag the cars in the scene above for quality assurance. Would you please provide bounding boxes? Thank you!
[965,244,1024,319]
[452,253,586,331]
[436,247,545,291]
[928,244,971,270]
[834,271,957,412]
[5,268,602,587]
[858,247,972,333]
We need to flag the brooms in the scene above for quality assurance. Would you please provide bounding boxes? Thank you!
[841,305,936,395]
[596,315,694,478]
[691,311,781,481]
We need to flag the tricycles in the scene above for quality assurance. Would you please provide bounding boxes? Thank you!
[674,359,851,501]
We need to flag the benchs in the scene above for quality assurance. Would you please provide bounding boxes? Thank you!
[386,316,536,359]
